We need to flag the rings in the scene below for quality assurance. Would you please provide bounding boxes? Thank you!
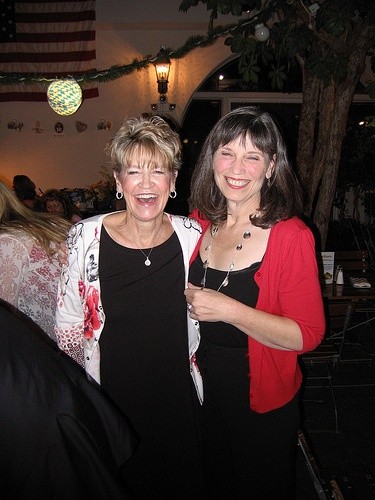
[186,303,193,309]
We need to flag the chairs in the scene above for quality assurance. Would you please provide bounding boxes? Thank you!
[300,298,357,434]
[327,249,375,363]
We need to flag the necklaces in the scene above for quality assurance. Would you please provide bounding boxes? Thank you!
[201,221,254,293]
[122,221,166,268]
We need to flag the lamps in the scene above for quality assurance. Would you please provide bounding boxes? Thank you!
[151,48,173,93]
[47,79,83,117]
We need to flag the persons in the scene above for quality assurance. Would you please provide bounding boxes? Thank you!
[0,174,84,373]
[183,105,325,500]
[41,188,84,222]
[0,296,153,500]
[12,174,41,211]
[53,112,201,500]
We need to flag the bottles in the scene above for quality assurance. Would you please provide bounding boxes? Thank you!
[335,266,345,285]
[360,251,369,279]
[334,264,340,281]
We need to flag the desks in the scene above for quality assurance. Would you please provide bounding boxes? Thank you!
[303,276,375,390]
